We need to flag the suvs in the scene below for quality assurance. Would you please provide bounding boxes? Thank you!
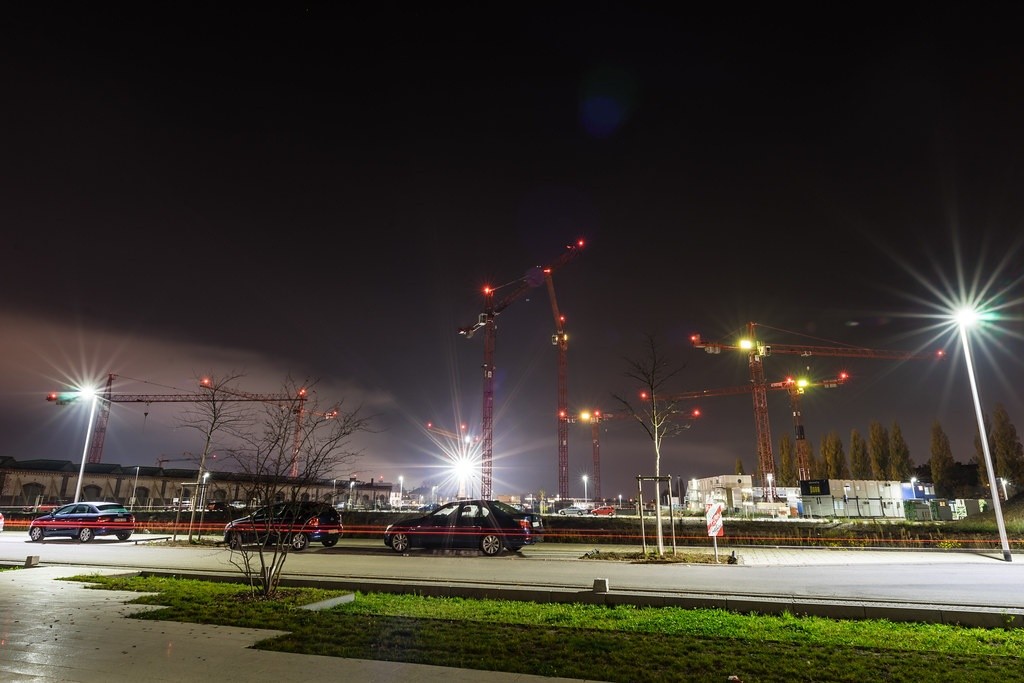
[223,502,343,549]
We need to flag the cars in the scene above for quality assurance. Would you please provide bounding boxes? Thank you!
[383,500,543,556]
[29,502,136,542]
[591,506,615,516]
[559,506,588,516]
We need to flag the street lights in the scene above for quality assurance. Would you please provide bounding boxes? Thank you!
[433,484,439,503]
[618,494,623,508]
[911,477,916,498]
[199,473,211,509]
[398,475,404,511]
[953,305,1013,564]
[131,466,140,511]
[74,384,98,502]
[843,486,850,518]
[582,475,589,504]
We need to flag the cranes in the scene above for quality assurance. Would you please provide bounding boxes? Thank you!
[426,240,701,500]
[639,321,944,504]
[44,372,307,463]
[197,376,341,477]
